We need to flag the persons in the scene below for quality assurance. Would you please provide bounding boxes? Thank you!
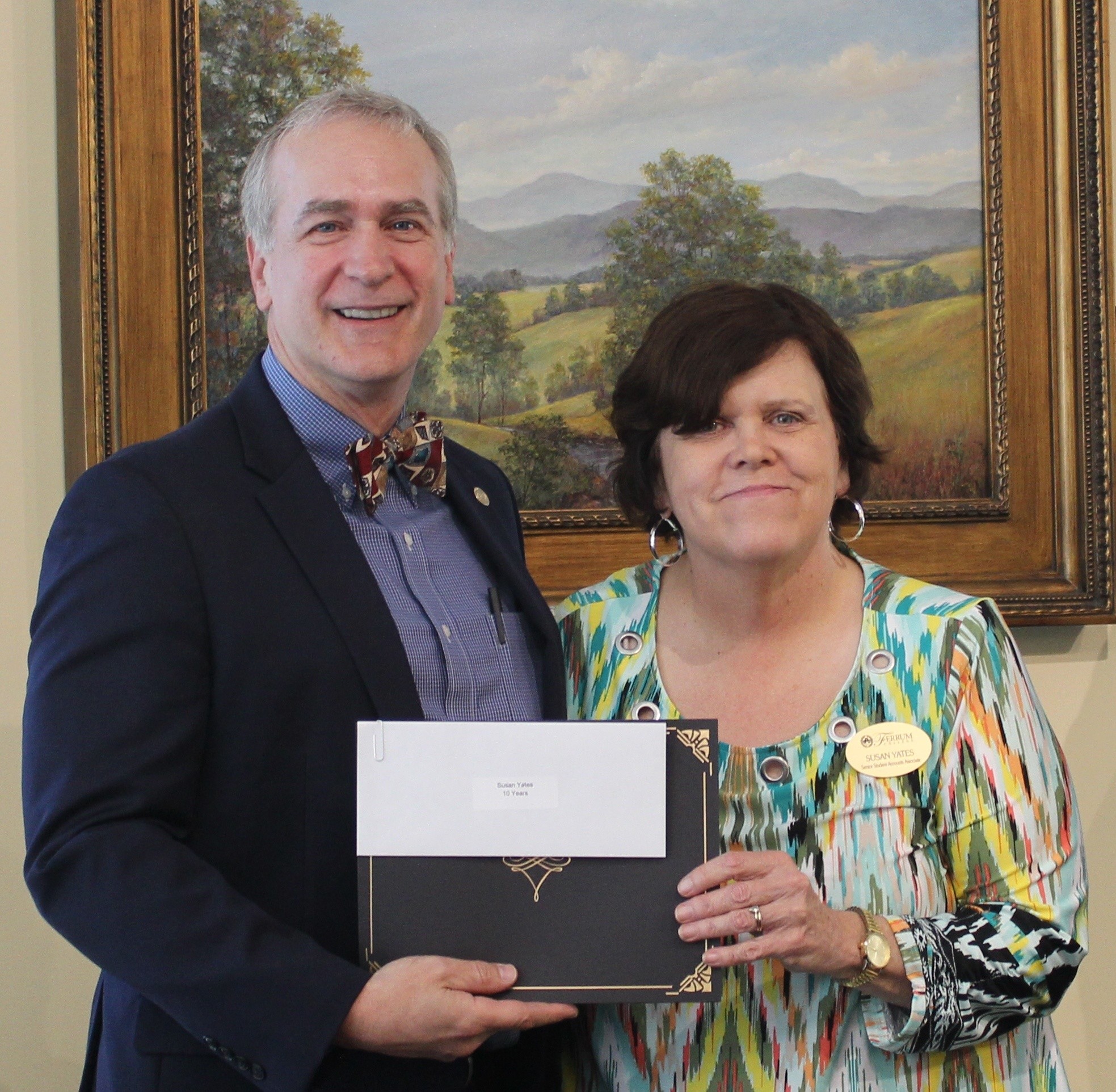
[544,280,1090,1090]
[20,85,581,1092]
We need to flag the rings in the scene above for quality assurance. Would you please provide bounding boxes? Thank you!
[749,905,762,934]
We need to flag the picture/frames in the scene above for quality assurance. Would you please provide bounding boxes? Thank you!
[73,0,1116,624]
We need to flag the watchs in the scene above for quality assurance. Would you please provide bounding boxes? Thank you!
[836,906,891,989]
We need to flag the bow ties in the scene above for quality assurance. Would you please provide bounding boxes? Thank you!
[344,410,447,516]
